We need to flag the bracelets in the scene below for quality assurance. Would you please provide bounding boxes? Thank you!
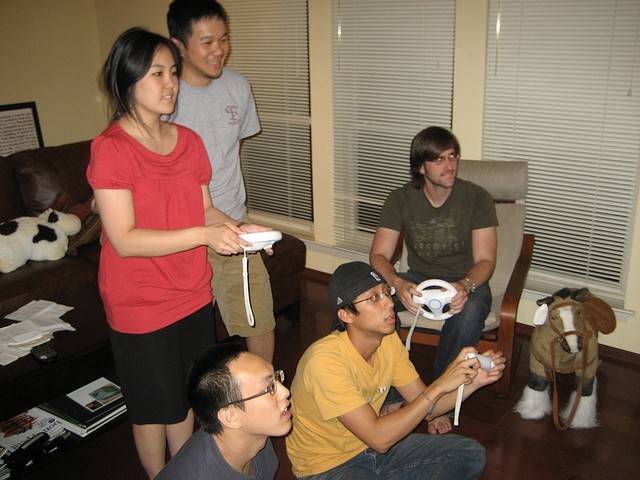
[418,391,436,415]
[462,276,477,294]
[237,220,245,227]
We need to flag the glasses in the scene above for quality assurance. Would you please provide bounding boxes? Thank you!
[351,286,396,305]
[434,151,460,167]
[223,368,285,409]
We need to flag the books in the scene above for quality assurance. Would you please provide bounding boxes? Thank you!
[0,446,11,480]
[36,370,128,438]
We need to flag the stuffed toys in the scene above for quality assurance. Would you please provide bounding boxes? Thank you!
[512,287,616,429]
[0,208,82,274]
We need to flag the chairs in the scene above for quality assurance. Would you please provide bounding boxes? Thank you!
[375,158,535,397]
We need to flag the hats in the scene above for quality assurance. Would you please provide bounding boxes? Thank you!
[328,262,386,310]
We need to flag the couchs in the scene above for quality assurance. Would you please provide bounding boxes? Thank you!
[0,138,307,326]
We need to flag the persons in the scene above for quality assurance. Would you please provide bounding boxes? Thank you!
[369,126,499,434]
[153,342,293,480]
[284,261,507,480]
[11,156,98,222]
[85,26,275,479]
[159,0,275,363]
